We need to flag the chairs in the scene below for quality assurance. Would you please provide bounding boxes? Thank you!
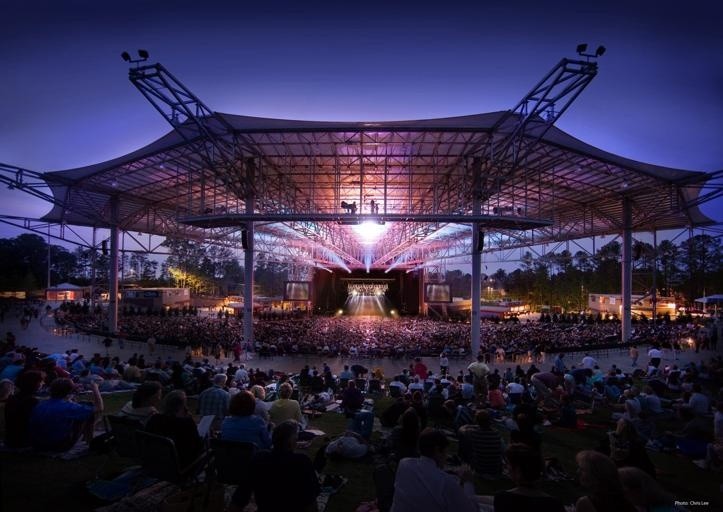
[350,412,375,437]
[411,388,423,394]
[388,386,402,398]
[209,438,259,485]
[136,429,215,510]
[508,393,521,402]
[101,414,145,448]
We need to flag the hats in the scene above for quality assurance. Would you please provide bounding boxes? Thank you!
[326,437,367,457]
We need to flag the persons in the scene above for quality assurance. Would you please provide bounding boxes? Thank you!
[0,296,723,512]
